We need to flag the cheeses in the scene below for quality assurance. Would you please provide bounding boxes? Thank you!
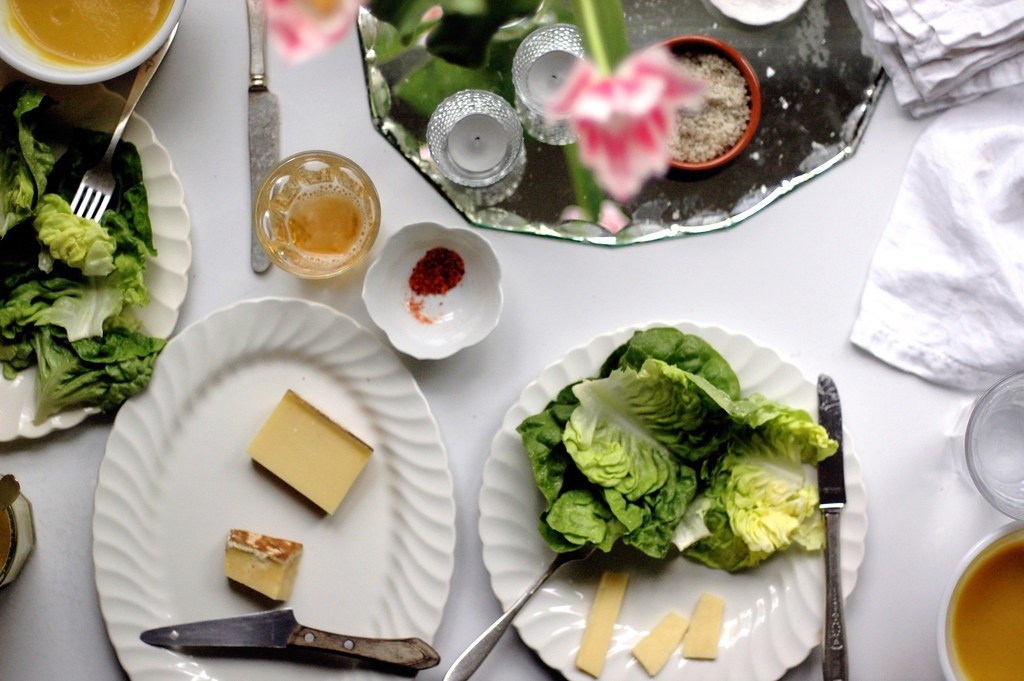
[249,389,373,515]
[225,527,304,602]
[576,569,725,679]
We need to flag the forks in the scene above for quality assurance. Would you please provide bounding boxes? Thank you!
[70,21,179,223]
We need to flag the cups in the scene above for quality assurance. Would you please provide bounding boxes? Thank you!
[426,89,523,187]
[948,370,1024,520]
[512,23,587,145]
[254,150,382,280]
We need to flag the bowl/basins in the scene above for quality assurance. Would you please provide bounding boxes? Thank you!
[935,520,1024,681]
[0,0,187,84]
[653,35,763,181]
[361,221,504,359]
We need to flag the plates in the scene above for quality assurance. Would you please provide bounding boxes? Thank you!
[92,298,458,681]
[477,322,868,681]
[0,82,193,446]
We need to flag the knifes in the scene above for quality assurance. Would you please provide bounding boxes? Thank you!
[816,373,849,681]
[247,1,281,273]
[141,609,441,670]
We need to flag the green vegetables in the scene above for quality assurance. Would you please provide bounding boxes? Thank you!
[517,328,827,570]
[0,87,165,425]
[366,0,630,221]
